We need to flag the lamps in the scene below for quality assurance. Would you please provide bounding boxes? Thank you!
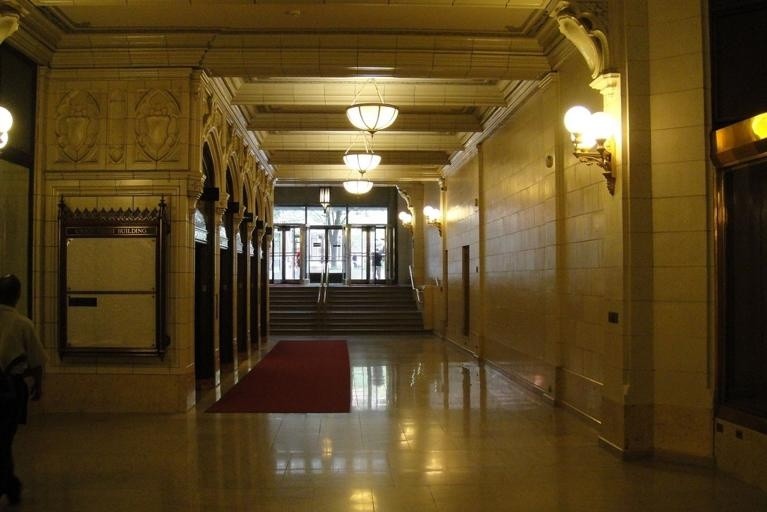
[342,130,382,174]
[424,205,442,237]
[346,78,401,138]
[399,211,414,233]
[0,105,13,151]
[565,106,616,196]
[343,168,373,196]
[319,187,331,212]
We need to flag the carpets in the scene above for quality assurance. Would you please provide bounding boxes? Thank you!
[205,340,352,413]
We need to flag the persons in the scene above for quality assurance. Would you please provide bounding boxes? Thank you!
[371,247,382,281]
[0,273,48,506]
[293,248,300,276]
